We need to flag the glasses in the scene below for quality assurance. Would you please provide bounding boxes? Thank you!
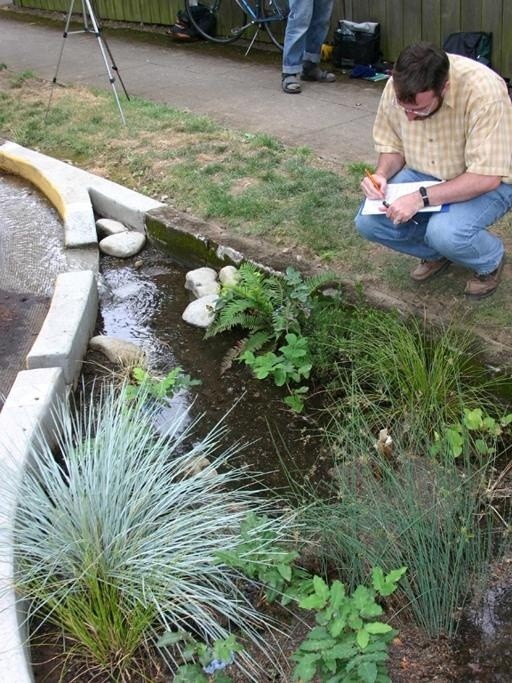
[390,88,441,117]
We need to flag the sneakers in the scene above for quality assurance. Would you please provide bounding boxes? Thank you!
[463,252,505,300]
[411,256,452,282]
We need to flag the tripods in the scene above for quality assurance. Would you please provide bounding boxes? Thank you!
[43,0,131,125]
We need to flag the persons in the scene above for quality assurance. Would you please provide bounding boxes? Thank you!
[356,38,511,299]
[281,0,337,94]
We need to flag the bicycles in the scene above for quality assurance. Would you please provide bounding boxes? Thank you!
[183,0,290,52]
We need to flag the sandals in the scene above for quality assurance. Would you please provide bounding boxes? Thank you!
[300,64,336,83]
[281,72,302,94]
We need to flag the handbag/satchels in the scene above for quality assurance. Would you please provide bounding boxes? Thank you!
[331,19,381,69]
[172,4,217,43]
[442,31,491,66]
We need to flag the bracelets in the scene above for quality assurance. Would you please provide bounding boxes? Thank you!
[419,186,429,207]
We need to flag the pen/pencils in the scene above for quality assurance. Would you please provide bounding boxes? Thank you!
[363,167,386,199]
[382,201,418,225]
